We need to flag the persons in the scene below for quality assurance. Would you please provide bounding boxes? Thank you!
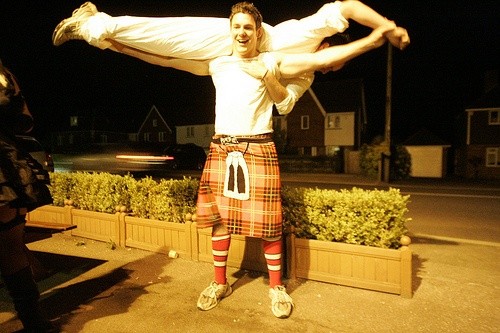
[105,4,396,319]
[53,0,410,115]
[0,58,46,333]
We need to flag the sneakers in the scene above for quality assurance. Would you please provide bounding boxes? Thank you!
[269,285,293,316]
[197,278,232,311]
[52,1,99,46]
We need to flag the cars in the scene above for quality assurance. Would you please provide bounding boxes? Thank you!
[14,135,54,173]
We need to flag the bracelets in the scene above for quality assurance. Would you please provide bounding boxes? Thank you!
[261,69,268,81]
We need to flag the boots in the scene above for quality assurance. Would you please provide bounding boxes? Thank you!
[3,265,60,333]
